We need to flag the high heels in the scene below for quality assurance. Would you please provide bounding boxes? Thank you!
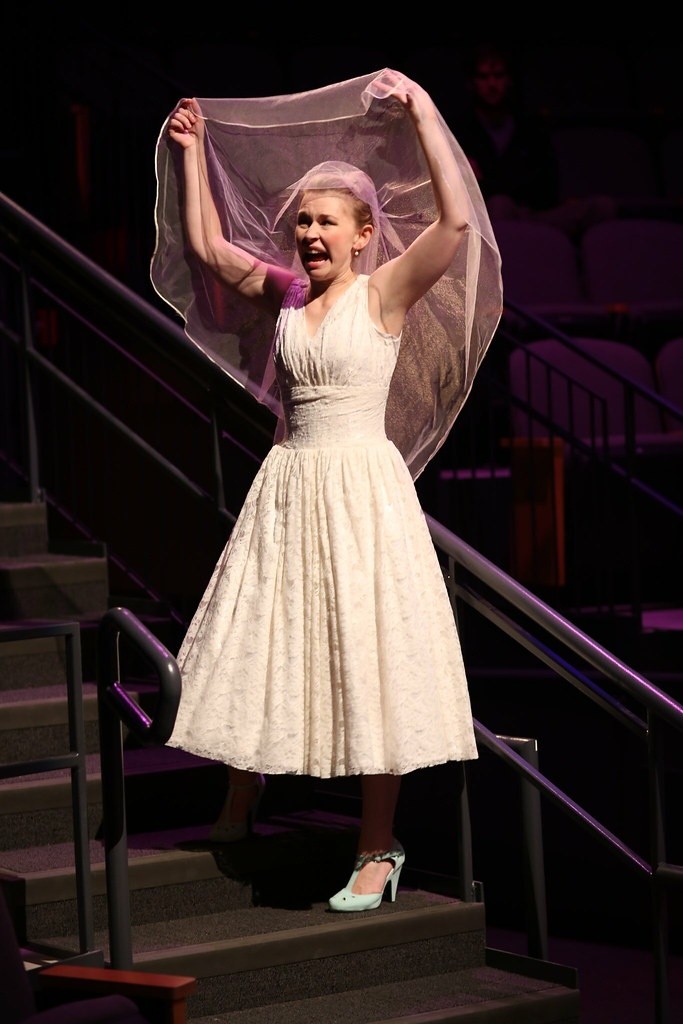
[209,772,266,843]
[328,842,405,914]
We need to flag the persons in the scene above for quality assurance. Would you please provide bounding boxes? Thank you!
[452,41,561,210]
[152,69,503,912]
